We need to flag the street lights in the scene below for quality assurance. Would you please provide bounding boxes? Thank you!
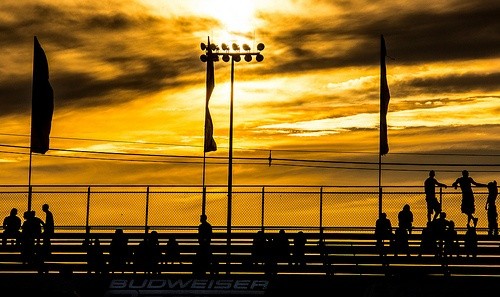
[200,42,265,275]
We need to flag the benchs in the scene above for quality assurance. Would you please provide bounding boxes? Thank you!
[0,224,500,287]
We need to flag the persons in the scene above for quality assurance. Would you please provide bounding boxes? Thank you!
[3,169,500,280]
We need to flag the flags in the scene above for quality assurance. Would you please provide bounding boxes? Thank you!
[204,37,217,154]
[30,36,54,153]
[379,34,391,156]
